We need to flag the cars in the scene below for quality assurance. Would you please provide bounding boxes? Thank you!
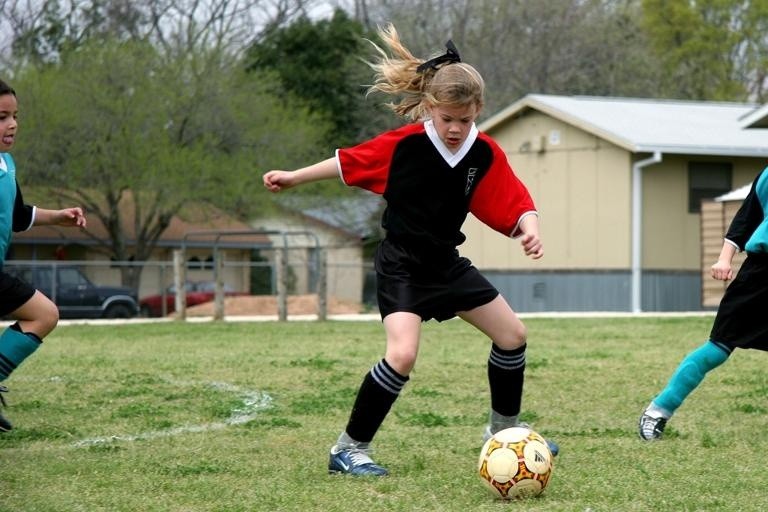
[136,279,252,318]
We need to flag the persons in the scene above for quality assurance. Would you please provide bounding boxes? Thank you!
[260,17,559,481]
[636,163,768,442]
[0,75,88,436]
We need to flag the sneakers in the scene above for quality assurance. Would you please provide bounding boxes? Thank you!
[0,386,13,432]
[482,423,558,457]
[327,441,387,479]
[639,409,667,441]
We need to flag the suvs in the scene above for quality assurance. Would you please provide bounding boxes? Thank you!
[1,264,142,321]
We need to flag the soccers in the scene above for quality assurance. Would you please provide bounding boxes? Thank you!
[477,427,553,499]
